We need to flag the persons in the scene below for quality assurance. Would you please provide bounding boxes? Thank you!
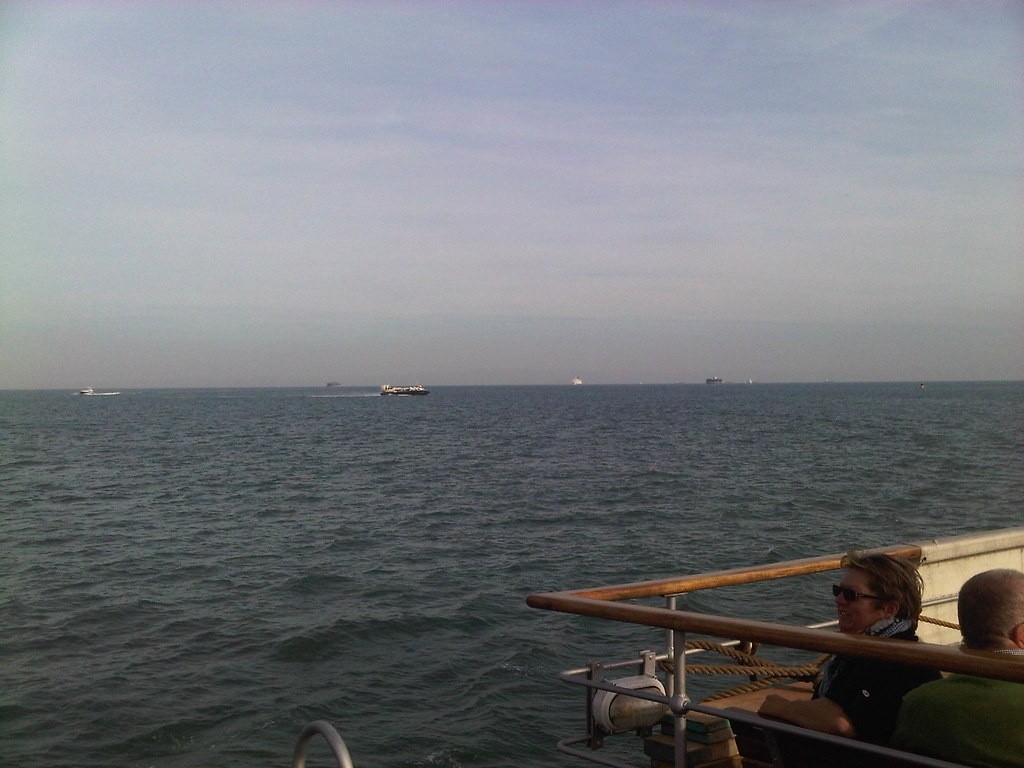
[889,568,1024,768]
[758,549,944,746]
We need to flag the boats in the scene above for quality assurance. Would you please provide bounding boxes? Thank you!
[380,384,430,396]
[79,386,121,397]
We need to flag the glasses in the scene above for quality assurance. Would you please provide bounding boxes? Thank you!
[832,584,883,602]
